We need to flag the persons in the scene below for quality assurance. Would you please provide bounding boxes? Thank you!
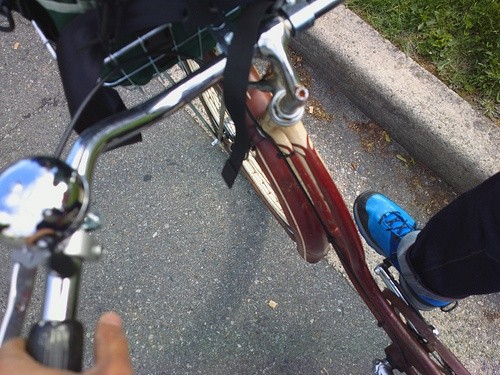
[0,170,500,375]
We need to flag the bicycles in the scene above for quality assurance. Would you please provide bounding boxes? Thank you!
[0,0,500,375]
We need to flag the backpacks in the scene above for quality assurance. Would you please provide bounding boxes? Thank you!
[0,0,303,155]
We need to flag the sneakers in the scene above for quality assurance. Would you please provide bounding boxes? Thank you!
[352,191,451,312]
